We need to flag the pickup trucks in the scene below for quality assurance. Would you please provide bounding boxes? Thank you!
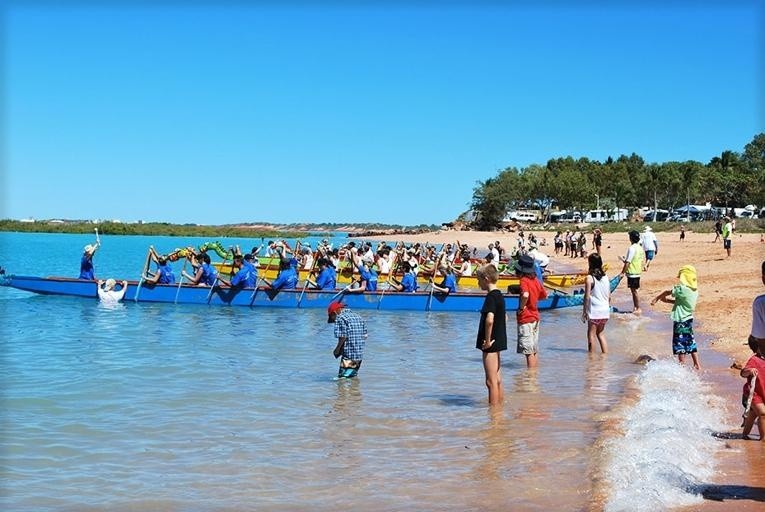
[555,216,582,223]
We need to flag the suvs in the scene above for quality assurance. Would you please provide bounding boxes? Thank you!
[510,213,538,224]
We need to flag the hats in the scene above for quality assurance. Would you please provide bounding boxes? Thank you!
[643,225,652,231]
[327,302,344,323]
[628,231,640,242]
[514,256,535,273]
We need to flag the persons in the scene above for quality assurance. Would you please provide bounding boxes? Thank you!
[651,265,701,369]
[80,238,101,281]
[750,260,765,358]
[553,229,602,259]
[514,256,548,369]
[618,230,647,318]
[640,225,659,271]
[141,231,548,295]
[741,335,765,441]
[679,225,685,243]
[582,252,611,354]
[96,279,128,310]
[710,217,736,259]
[475,264,509,403]
[327,302,368,379]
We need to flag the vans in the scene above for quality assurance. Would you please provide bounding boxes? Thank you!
[644,212,666,222]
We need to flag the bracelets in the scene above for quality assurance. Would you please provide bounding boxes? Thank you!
[519,309,523,310]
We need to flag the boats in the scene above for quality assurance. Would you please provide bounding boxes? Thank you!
[168,247,609,290]
[198,241,511,275]
[0,267,625,311]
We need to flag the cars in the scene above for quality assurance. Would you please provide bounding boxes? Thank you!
[669,214,681,222]
[677,214,695,222]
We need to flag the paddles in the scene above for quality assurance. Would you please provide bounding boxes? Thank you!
[428,256,440,311]
[377,256,400,310]
[328,275,362,304]
[174,255,188,306]
[134,248,151,302]
[249,252,275,304]
[206,248,231,301]
[297,252,319,305]
